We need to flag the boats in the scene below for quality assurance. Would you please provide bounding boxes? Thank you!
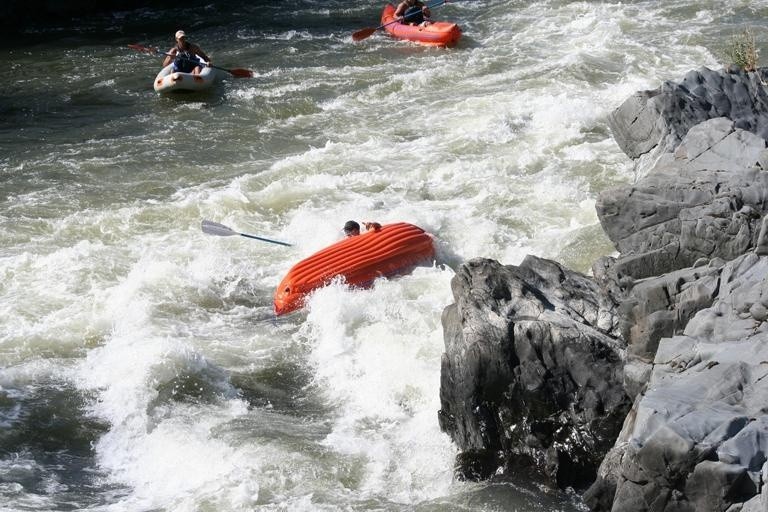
[151,53,219,96]
[269,221,437,318]
[379,1,463,47]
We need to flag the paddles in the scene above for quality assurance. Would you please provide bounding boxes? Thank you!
[127,43,253,78]
[201,220,295,247]
[353,1,449,41]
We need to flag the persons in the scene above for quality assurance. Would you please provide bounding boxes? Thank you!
[162,30,213,76]
[392,0,432,31]
[342,221,382,238]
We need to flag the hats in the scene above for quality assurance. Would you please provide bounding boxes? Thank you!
[343,221,359,231]
[175,30,185,39]
